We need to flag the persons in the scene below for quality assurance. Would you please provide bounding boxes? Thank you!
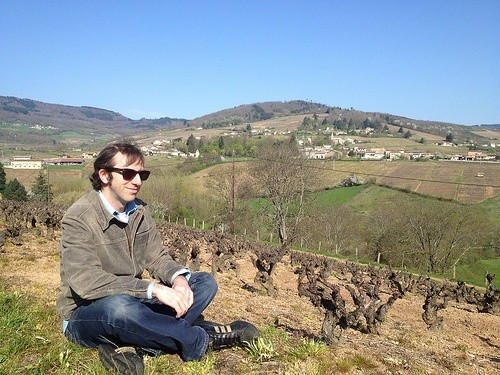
[55,142,260,375]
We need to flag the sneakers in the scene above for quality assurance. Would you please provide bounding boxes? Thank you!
[194,321,259,350]
[98,344,144,375]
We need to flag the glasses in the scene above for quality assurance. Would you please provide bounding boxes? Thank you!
[106,168,151,181]
